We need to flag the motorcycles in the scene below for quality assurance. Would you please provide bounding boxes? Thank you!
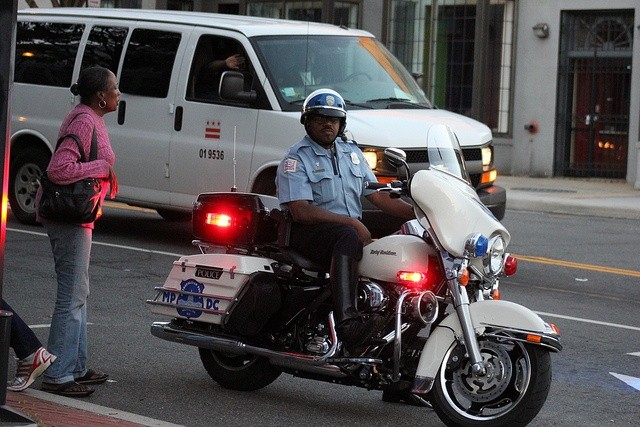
[144,123,563,427]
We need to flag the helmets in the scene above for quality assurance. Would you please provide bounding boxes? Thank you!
[299,87,347,125]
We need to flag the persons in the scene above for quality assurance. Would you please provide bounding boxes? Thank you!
[276,88,415,352]
[290,48,320,87]
[208,52,245,71]
[1,298,57,392]
[40,66,121,396]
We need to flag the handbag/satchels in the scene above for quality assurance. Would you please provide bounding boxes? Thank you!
[38,112,101,225]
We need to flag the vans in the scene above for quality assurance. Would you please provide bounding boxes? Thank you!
[7,8,507,244]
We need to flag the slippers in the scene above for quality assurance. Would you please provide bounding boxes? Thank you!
[36,382,94,397]
[79,368,109,382]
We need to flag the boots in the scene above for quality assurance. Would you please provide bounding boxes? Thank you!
[329,253,388,352]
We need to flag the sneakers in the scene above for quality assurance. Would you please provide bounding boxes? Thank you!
[7,347,58,393]
